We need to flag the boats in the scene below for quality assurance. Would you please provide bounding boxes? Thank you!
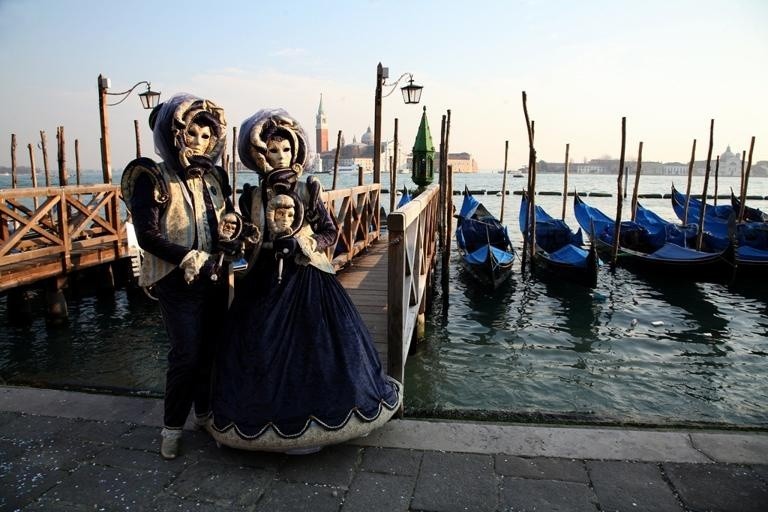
[569,188,734,281]
[327,164,362,175]
[519,189,599,294]
[457,186,515,291]
[668,182,767,246]
[511,172,523,178]
[635,199,767,282]
[396,185,412,209]
[368,206,387,234]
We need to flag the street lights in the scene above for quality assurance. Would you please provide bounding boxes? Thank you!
[97,74,161,186]
[372,59,424,189]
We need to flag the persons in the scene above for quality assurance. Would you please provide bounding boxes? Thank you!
[205,109,404,456]
[120,91,245,460]
[267,194,296,234]
